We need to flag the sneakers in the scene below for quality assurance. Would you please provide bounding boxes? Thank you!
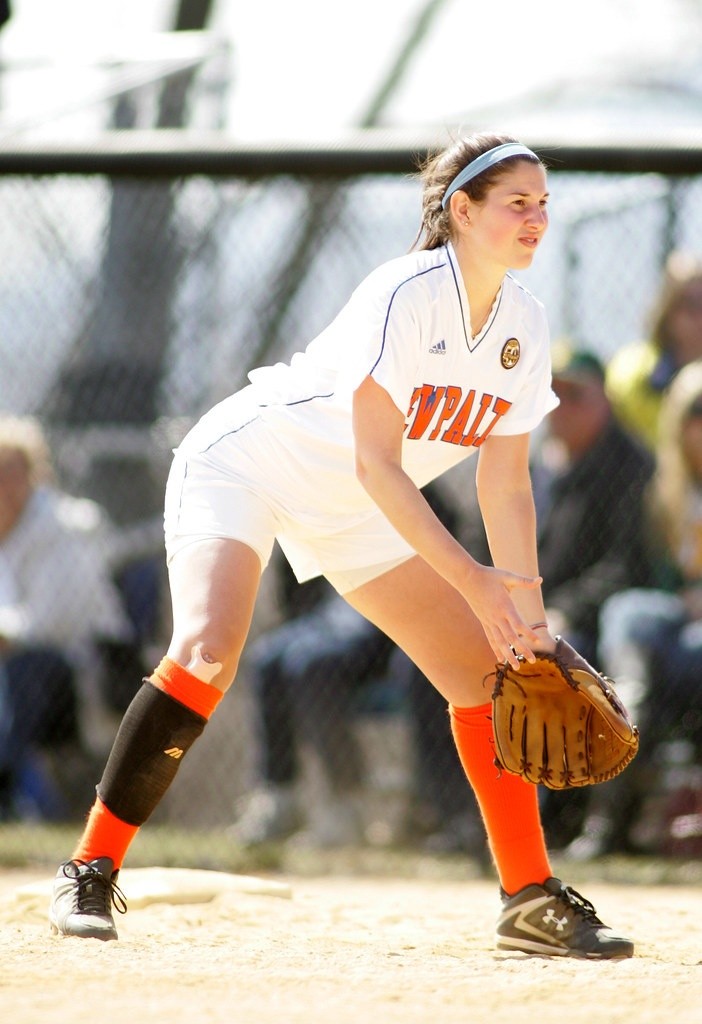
[48,857,127,941]
[493,878,635,958]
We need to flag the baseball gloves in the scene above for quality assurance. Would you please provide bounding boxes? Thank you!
[482,635,641,791]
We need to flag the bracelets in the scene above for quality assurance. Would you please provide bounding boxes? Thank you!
[516,620,549,638]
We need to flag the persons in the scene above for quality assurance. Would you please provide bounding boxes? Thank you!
[0,429,136,827]
[218,250,702,871]
[42,129,637,967]
